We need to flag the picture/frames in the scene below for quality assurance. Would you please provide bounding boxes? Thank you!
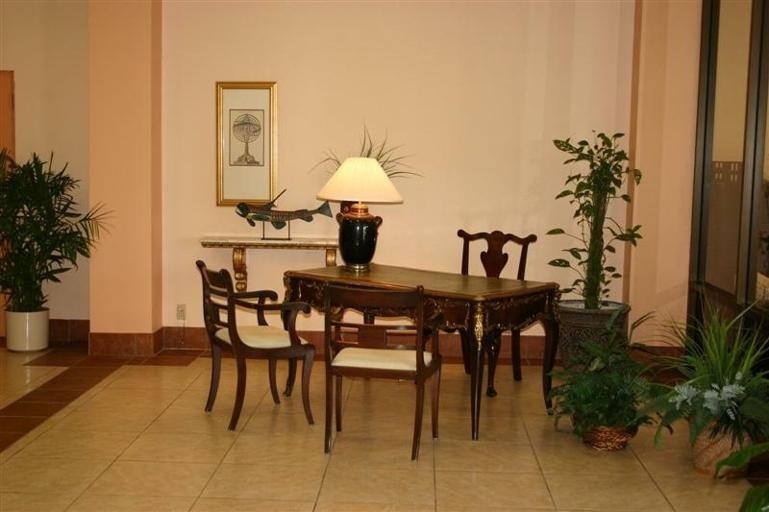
[215,80,279,208]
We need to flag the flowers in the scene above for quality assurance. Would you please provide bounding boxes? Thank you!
[616,274,769,454]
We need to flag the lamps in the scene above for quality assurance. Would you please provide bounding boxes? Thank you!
[315,156,405,273]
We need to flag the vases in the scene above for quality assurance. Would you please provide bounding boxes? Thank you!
[690,416,755,477]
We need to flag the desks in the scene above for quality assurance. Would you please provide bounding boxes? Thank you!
[281,260,563,441]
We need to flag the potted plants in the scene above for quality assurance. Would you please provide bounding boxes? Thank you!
[0,145,119,354]
[545,130,643,374]
[542,304,659,455]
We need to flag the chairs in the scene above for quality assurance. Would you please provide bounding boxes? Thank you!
[320,280,444,465]
[459,228,537,399]
[195,259,318,432]
